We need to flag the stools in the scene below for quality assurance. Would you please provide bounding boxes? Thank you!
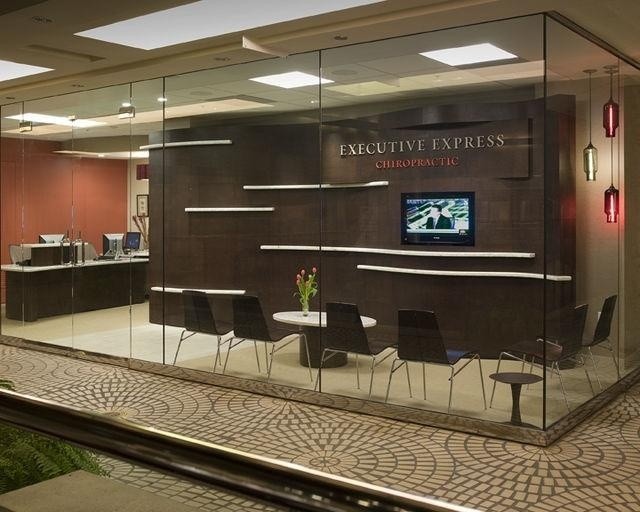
[488,372,543,431]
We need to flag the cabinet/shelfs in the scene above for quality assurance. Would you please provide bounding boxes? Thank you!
[0,258,149,323]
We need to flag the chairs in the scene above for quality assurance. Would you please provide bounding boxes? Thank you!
[172,291,234,366]
[312,299,412,401]
[529,293,620,393]
[486,303,595,412]
[384,306,488,414]
[220,294,314,385]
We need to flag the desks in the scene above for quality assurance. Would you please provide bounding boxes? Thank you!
[273,308,377,369]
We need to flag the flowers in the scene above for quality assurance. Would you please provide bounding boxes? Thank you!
[292,267,319,305]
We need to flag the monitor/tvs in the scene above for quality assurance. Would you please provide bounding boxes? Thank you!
[39,234,65,243]
[103,233,125,261]
[400,191,475,247]
[125,232,141,254]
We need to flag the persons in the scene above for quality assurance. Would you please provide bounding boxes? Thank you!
[426,204,452,229]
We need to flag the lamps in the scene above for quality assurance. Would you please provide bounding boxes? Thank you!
[583,64,621,222]
